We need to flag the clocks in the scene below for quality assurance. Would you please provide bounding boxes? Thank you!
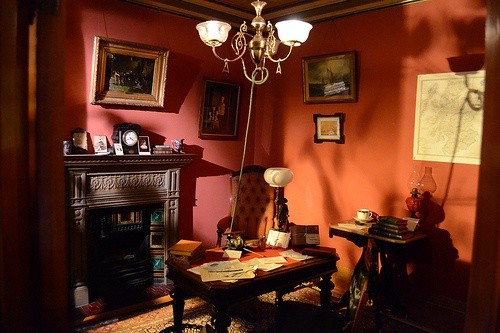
[111,123,140,154]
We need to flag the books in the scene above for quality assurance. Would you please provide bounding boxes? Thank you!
[169,239,205,261]
[368,216,415,241]
[151,145,173,154]
[304,246,337,255]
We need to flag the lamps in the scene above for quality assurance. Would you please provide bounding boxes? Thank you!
[264,167,294,231]
[405,170,423,231]
[196,0,313,74]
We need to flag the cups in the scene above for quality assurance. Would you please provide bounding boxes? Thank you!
[357,209,373,220]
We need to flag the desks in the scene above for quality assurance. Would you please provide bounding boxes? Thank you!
[165,240,340,333]
[329,219,426,307]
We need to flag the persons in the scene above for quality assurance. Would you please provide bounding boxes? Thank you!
[216,96,226,127]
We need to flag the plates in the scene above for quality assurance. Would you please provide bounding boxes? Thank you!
[353,216,374,223]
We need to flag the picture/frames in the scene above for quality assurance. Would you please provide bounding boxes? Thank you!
[197,78,242,141]
[302,51,357,103]
[313,112,344,143]
[89,36,170,108]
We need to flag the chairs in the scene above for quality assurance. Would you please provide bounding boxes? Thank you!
[216,165,281,248]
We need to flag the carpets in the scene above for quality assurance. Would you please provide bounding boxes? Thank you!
[82,285,341,333]
